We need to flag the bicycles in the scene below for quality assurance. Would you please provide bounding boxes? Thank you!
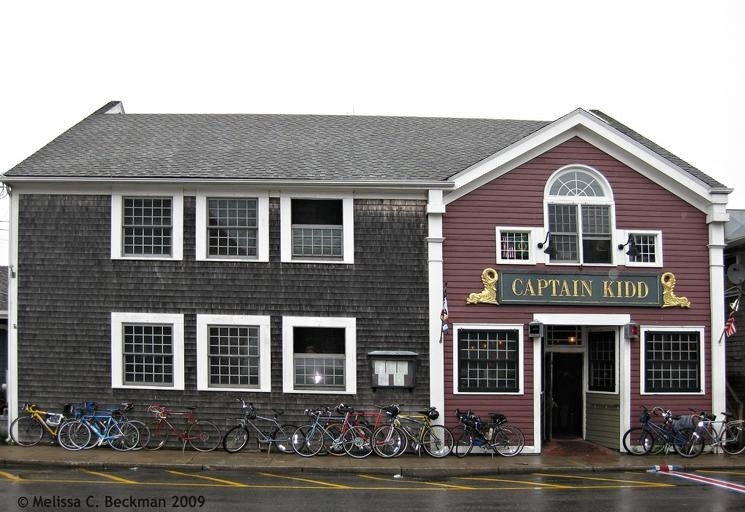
[619,403,745,457]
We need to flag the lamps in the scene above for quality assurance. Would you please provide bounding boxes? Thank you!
[537,232,560,256]
[618,232,641,257]
